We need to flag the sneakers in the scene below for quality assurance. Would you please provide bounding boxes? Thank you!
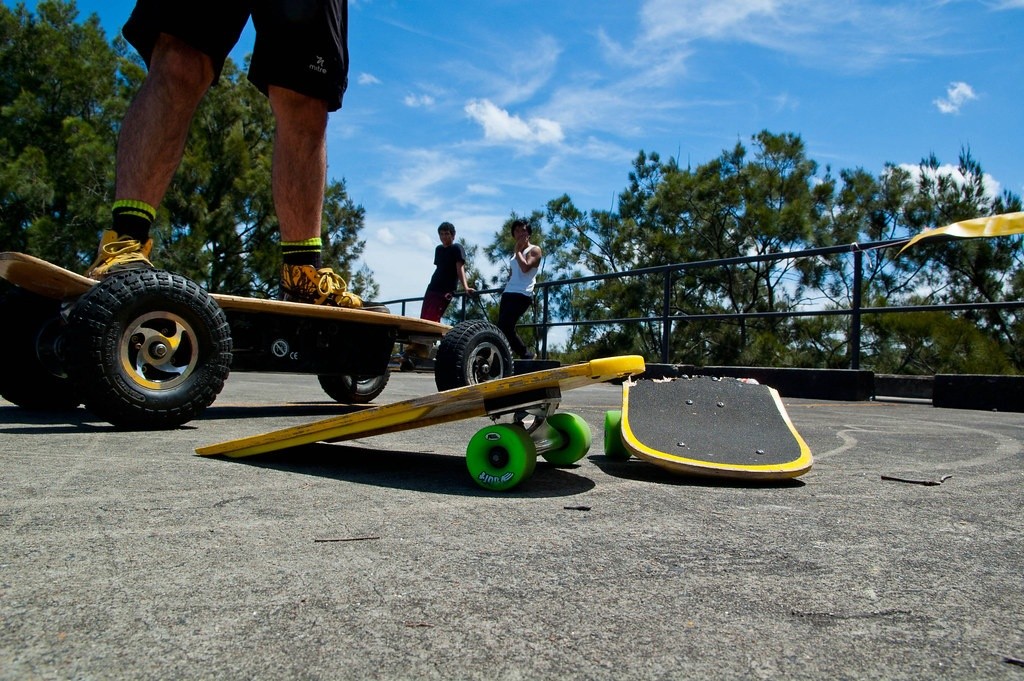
[277,263,363,308]
[85,229,154,279]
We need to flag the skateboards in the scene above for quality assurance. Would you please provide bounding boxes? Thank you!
[0,251,513,428]
[193,354,814,490]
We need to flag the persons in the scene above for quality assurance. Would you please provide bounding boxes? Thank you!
[498,219,542,358]
[406,222,476,357]
[85,0,362,307]
